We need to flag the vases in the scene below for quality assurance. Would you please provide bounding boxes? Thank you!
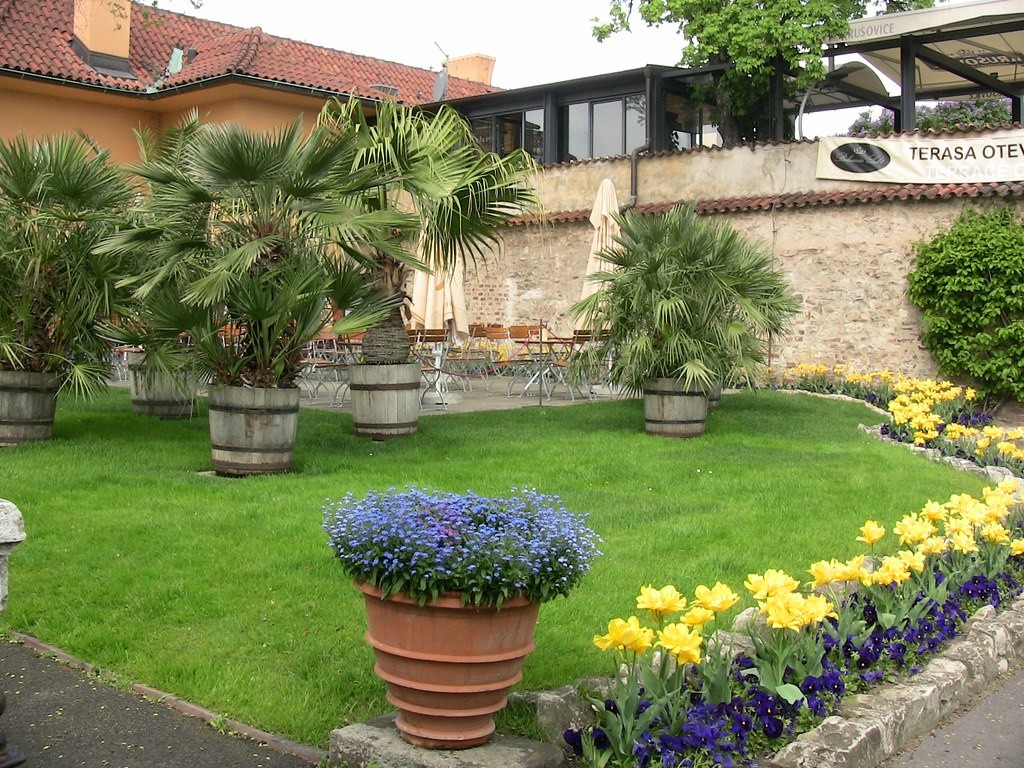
[352,573,541,750]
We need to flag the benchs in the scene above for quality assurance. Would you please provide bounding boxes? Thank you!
[294,322,614,408]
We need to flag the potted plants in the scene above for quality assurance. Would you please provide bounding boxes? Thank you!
[563,199,805,440]
[0,98,546,476]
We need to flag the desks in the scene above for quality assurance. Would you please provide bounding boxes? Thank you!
[516,339,584,401]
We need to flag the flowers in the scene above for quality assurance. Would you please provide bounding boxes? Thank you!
[320,484,607,605]
